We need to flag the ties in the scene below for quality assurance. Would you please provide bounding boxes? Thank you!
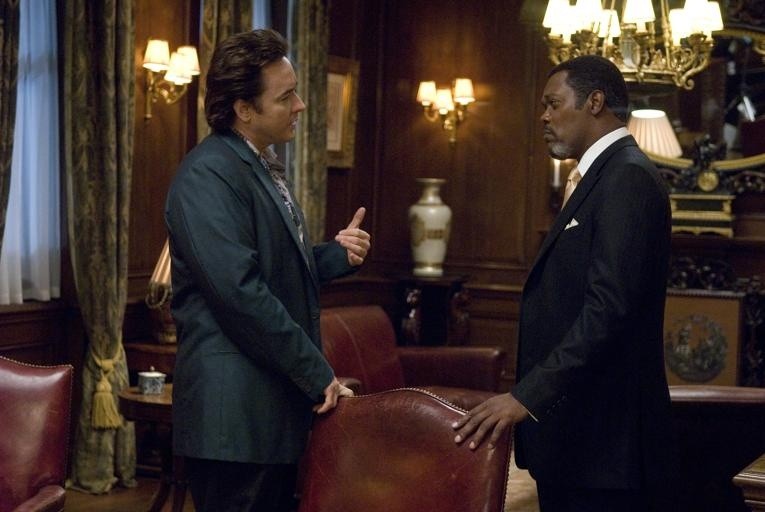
[562,164,583,210]
[259,153,302,244]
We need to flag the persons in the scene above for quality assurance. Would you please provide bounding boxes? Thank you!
[450,55,675,512]
[164,26,372,512]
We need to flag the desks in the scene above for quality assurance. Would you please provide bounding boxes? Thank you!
[386,264,472,347]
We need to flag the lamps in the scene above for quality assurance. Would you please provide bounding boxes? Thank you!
[542,0,724,89]
[417,78,475,144]
[147,237,177,345]
[627,109,683,159]
[141,40,202,119]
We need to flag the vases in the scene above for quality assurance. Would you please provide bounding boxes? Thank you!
[409,176,453,278]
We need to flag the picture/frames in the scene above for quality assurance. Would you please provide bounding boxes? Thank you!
[327,55,360,169]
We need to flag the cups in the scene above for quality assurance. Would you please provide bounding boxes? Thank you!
[137,366,167,396]
